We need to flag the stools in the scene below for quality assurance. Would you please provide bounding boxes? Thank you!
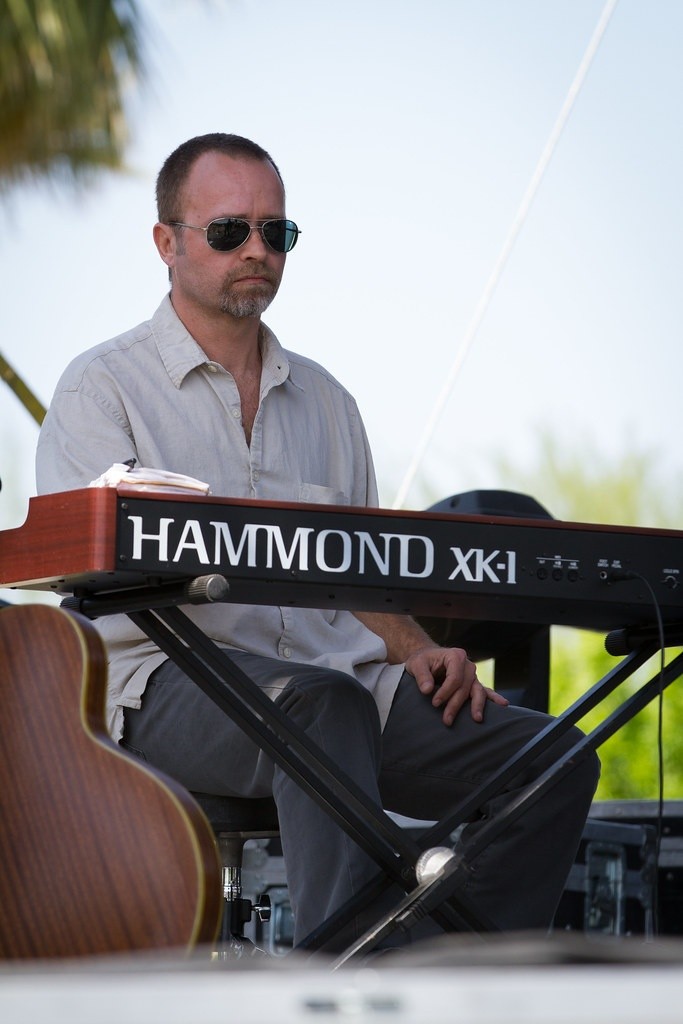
[167,791,299,962]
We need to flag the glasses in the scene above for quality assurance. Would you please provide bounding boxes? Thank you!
[165,218,301,254]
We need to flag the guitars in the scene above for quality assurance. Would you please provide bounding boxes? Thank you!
[1,604,221,967]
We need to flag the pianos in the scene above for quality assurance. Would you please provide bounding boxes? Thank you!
[1,487,683,630]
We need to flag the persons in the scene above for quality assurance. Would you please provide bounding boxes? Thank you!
[35,132,602,962]
